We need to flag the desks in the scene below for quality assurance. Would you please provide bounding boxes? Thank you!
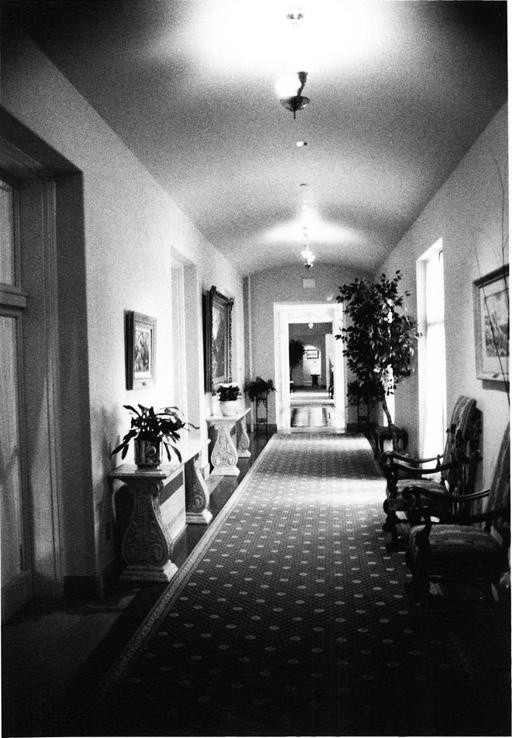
[106,438,211,584]
[206,407,252,477]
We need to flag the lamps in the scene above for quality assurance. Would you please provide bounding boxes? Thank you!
[275,53,313,120]
[301,245,316,273]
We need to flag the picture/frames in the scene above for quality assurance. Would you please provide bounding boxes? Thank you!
[127,310,158,390]
[205,285,234,392]
[472,263,509,381]
[306,349,319,359]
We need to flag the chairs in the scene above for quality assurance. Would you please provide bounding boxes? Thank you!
[403,420,510,614]
[377,395,483,551]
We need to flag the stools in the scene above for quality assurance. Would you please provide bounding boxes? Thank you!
[375,425,408,453]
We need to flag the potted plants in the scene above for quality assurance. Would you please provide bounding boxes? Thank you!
[217,385,241,416]
[112,404,201,469]
[333,269,423,480]
[243,376,276,402]
[345,380,382,409]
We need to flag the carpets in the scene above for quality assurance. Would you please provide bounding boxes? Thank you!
[65,432,510,734]
